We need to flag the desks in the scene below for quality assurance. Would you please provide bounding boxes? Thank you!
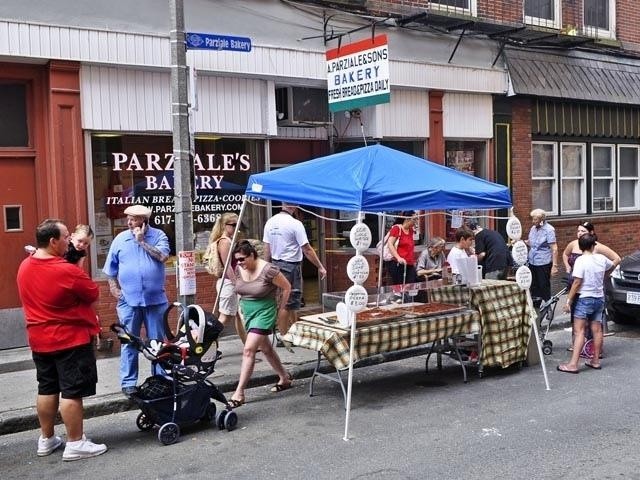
[426,278,550,391]
[282,302,481,443]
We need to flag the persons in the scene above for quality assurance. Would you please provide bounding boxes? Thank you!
[558,219,621,372]
[16,218,108,463]
[414,237,448,279]
[262,202,328,347]
[524,209,559,321]
[445,217,512,287]
[225,240,292,410]
[380,210,419,285]
[101,205,171,397]
[203,213,263,361]
[60,222,94,270]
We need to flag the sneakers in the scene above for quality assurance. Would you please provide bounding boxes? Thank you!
[36,435,62,457]
[62,435,108,461]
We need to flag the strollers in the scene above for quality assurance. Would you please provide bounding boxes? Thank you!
[511,276,575,353]
[109,301,237,446]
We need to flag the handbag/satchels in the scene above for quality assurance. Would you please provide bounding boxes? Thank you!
[376,232,399,261]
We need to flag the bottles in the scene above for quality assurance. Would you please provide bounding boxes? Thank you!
[442,265,462,285]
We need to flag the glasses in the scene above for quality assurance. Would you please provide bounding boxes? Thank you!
[226,222,237,227]
[234,255,249,262]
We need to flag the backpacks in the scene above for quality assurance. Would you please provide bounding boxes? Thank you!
[202,237,232,278]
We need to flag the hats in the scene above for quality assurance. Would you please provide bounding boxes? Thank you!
[124,205,152,219]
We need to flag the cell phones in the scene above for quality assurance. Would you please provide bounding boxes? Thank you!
[140,219,149,230]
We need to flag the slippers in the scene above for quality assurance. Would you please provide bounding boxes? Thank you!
[557,363,578,373]
[225,398,246,409]
[585,359,601,370]
[271,382,292,392]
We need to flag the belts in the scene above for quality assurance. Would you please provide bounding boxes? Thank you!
[271,259,301,264]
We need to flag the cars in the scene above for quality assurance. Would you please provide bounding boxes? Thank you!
[605,236,640,329]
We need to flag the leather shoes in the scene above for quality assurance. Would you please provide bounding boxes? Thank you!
[277,337,297,347]
[122,386,137,398]
